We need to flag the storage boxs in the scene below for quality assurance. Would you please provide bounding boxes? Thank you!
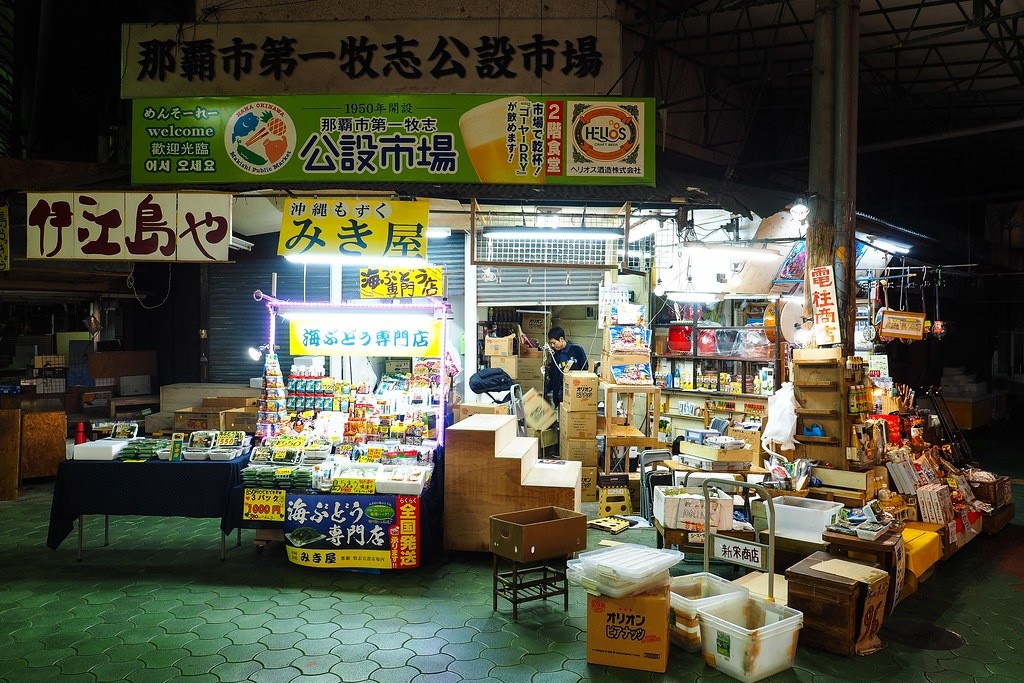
[173,396,260,432]
[586,577,671,674]
[967,475,1012,512]
[886,460,955,524]
[727,415,771,468]
[489,505,588,564]
[459,307,669,503]
[34,355,64,368]
[653,486,733,531]
[375,466,427,495]
[20,378,65,394]
[670,571,804,683]
[764,495,845,544]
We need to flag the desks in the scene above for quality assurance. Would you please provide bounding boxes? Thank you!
[47,451,249,559]
[664,459,771,496]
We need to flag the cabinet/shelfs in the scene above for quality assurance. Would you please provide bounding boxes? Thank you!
[651,353,786,448]
[792,348,848,471]
[596,383,662,476]
[490,552,568,622]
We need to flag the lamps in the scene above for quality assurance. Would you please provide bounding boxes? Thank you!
[247,343,269,361]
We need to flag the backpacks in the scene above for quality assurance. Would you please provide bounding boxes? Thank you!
[469,367,514,394]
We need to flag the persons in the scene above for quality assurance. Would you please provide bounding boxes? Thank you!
[540,327,588,409]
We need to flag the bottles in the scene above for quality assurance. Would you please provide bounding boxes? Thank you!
[390,420,405,445]
[846,356,874,413]
[910,419,925,438]
[851,426,861,453]
[488,308,516,321]
[878,484,899,507]
[661,485,688,498]
[753,375,760,395]
[673,364,680,388]
[349,408,367,422]
[490,322,510,337]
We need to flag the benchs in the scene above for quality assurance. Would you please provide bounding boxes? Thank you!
[107,395,160,421]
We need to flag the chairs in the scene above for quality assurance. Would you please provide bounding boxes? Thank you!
[640,448,675,527]
